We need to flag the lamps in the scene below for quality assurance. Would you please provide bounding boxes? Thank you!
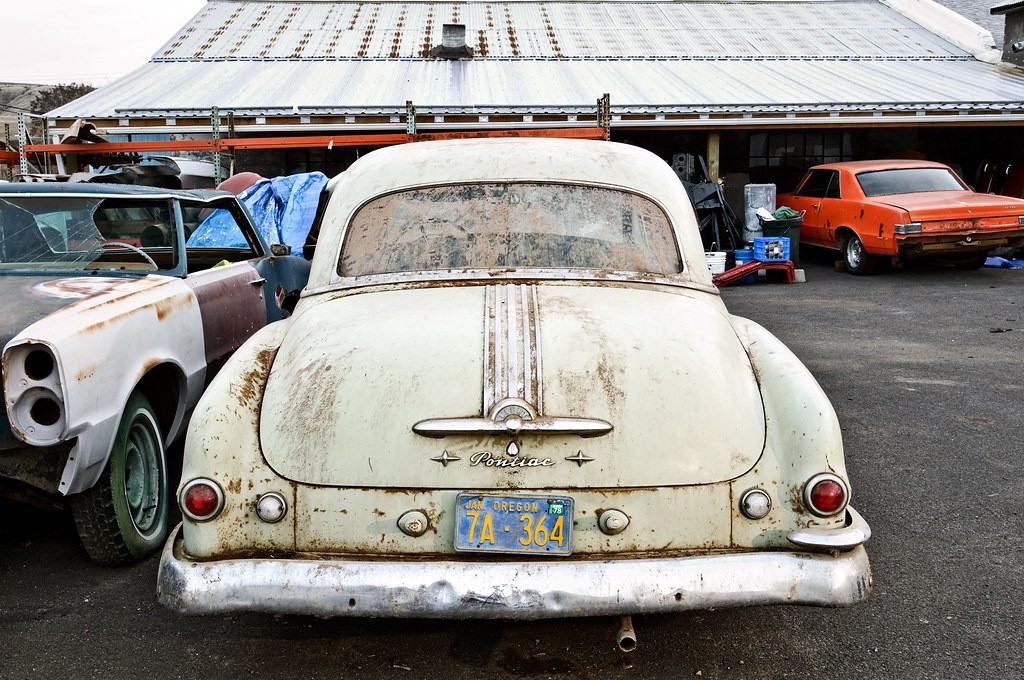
[327,138,334,150]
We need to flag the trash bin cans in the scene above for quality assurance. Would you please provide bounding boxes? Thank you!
[755,210,806,284]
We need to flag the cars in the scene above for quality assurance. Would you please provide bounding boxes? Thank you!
[0,179,311,571]
[152,134,876,652]
[775,158,1024,277]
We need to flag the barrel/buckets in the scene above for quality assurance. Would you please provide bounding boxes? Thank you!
[734,241,759,284]
[704,242,727,278]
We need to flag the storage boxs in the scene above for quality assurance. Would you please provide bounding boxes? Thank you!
[753,237,789,263]
